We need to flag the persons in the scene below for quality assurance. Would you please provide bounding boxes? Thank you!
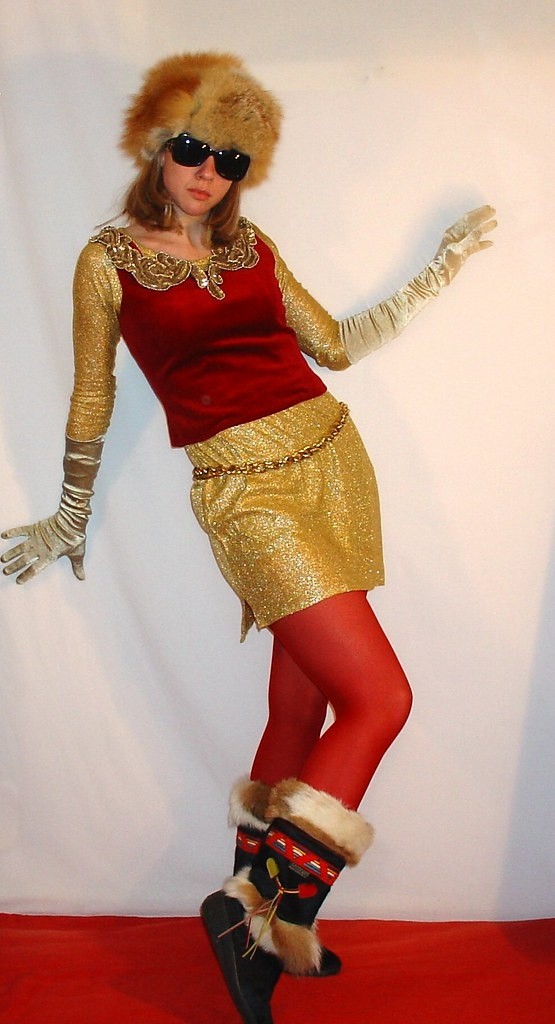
[0,50,496,1024]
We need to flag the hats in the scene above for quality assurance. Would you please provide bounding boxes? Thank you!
[120,50,282,190]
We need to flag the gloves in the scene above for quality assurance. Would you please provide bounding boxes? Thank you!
[0,435,107,586]
[339,204,498,364]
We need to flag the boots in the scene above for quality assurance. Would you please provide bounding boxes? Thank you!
[228,774,343,978]
[199,779,375,1024]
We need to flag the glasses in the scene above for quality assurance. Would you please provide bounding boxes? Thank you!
[163,133,252,182]
